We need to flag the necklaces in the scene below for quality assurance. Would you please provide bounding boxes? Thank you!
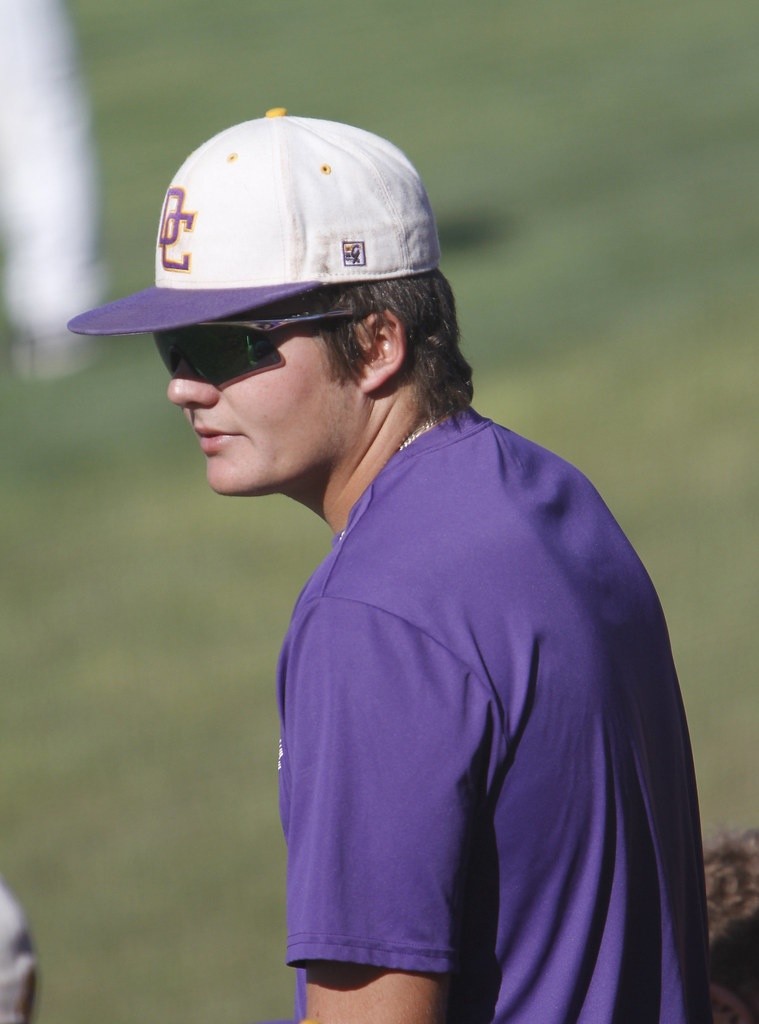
[337,402,455,541]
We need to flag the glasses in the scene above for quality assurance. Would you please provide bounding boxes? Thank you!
[152,312,353,388]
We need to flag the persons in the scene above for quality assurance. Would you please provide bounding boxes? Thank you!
[702,829,759,1024]
[0,0,101,384]
[149,107,714,1024]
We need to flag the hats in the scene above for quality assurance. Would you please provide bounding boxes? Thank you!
[66,108,440,333]
